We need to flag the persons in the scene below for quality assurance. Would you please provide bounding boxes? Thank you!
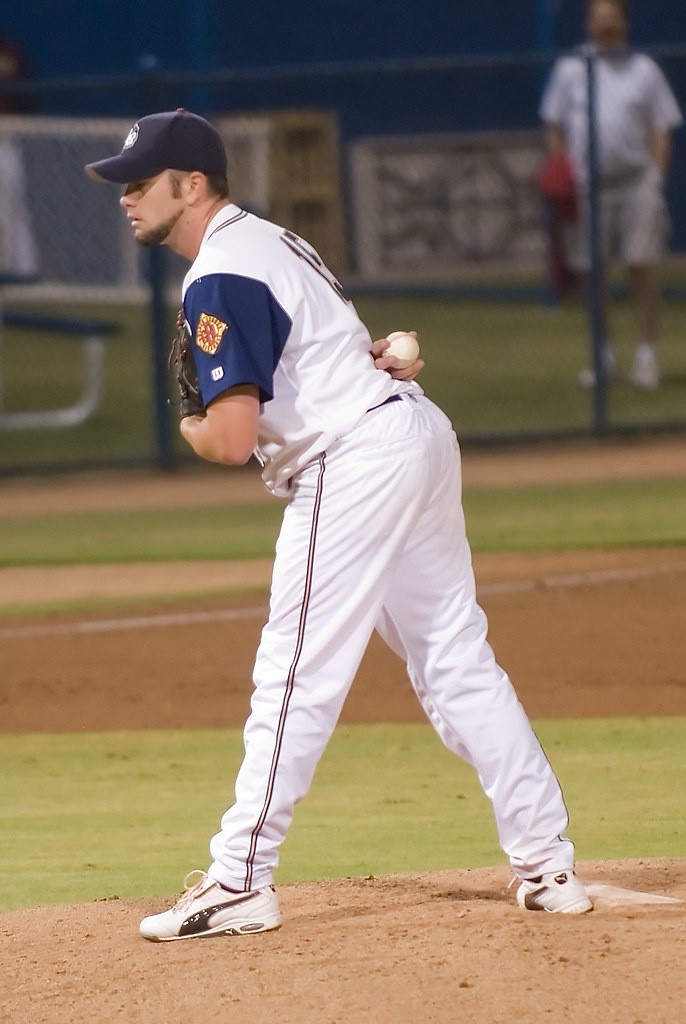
[87,109,592,944]
[541,0,682,392]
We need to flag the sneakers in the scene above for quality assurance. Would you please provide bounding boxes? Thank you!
[138,874,283,946]
[516,869,595,918]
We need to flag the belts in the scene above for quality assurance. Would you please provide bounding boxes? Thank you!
[364,390,423,413]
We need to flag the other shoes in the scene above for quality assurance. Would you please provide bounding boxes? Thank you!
[630,346,659,395]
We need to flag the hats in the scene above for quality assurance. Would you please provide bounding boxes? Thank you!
[82,108,230,185]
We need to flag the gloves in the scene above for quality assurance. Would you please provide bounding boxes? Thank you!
[170,330,202,421]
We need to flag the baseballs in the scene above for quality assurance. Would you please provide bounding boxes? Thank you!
[381,332,419,369]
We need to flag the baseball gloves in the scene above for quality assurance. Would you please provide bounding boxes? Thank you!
[174,308,203,418]
